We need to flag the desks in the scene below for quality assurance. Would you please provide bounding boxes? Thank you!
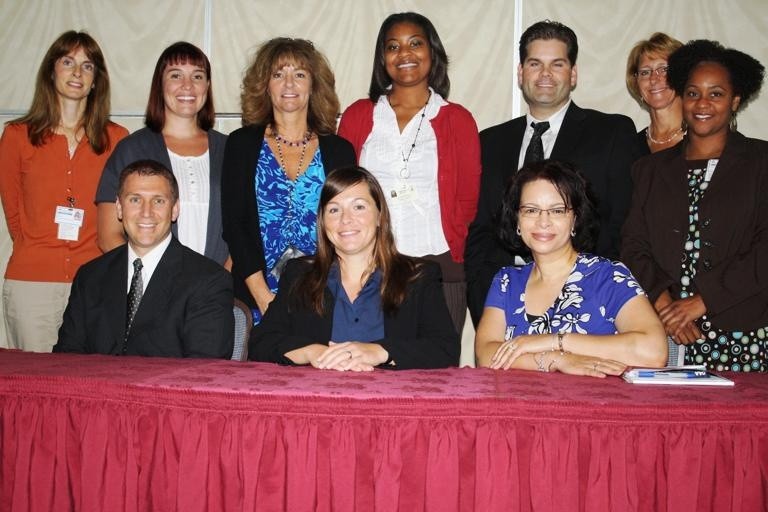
[0,351,768,512]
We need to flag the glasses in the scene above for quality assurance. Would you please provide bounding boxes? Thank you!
[520,205,572,216]
[636,66,668,77]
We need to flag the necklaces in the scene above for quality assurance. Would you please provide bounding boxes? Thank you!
[346,351,353,358]
[272,131,312,147]
[386,98,429,179]
[276,140,305,217]
[63,130,82,153]
[645,126,685,145]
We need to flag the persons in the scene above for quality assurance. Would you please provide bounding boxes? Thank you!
[621,40,768,374]
[51,159,237,357]
[0,32,129,353]
[473,162,669,378]
[626,30,687,153]
[95,42,234,270]
[337,13,482,328]
[222,37,357,317]
[248,167,462,371]
[465,19,638,331]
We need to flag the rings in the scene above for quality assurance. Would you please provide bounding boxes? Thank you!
[508,344,515,350]
[592,363,599,370]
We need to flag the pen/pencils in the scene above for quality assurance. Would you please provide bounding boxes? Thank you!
[634,371,708,377]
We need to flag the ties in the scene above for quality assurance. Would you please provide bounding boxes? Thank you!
[123,258,143,353]
[524,121,550,164]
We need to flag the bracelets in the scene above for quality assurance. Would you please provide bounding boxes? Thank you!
[383,353,393,366]
[533,350,571,373]
[552,331,565,352]
